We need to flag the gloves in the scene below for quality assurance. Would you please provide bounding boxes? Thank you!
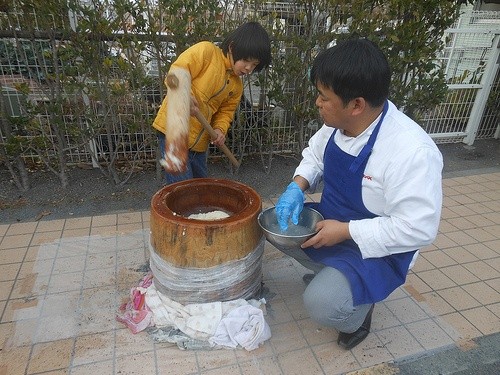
[275,181,307,233]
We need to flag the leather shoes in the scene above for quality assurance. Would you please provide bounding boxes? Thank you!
[303,273,315,285]
[338,301,375,351]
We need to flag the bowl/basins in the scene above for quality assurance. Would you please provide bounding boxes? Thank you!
[257,206,325,250]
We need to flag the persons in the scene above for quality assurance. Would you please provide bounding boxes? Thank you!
[149,22,271,188]
[264,38,443,351]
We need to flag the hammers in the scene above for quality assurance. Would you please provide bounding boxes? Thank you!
[160,67,241,177]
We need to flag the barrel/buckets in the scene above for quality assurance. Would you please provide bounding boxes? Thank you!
[150,177,262,303]
